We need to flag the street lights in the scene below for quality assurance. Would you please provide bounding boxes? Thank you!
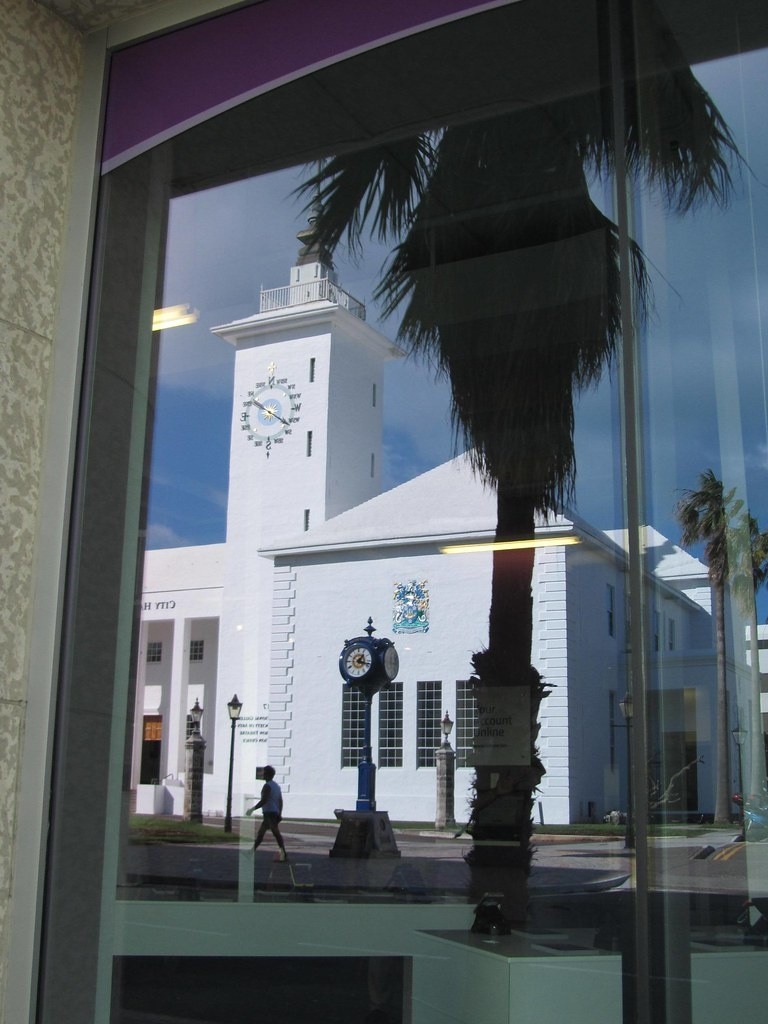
[732,723,748,842]
[618,689,636,850]
[223,693,244,834]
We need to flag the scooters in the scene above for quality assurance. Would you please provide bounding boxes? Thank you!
[732,775,768,844]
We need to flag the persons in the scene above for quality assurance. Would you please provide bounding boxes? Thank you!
[246,766,288,863]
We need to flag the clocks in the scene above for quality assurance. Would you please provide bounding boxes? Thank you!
[342,642,376,680]
[379,645,399,681]
[238,375,304,453]
